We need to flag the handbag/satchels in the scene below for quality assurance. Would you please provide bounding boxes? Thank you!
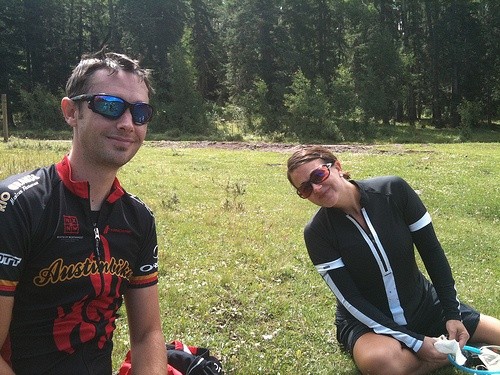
[116,340,223,375]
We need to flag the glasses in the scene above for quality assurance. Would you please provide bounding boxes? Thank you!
[295,161,333,199]
[70,93,153,126]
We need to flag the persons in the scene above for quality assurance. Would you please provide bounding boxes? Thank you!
[0,49,170,375]
[287,146,500,375]
